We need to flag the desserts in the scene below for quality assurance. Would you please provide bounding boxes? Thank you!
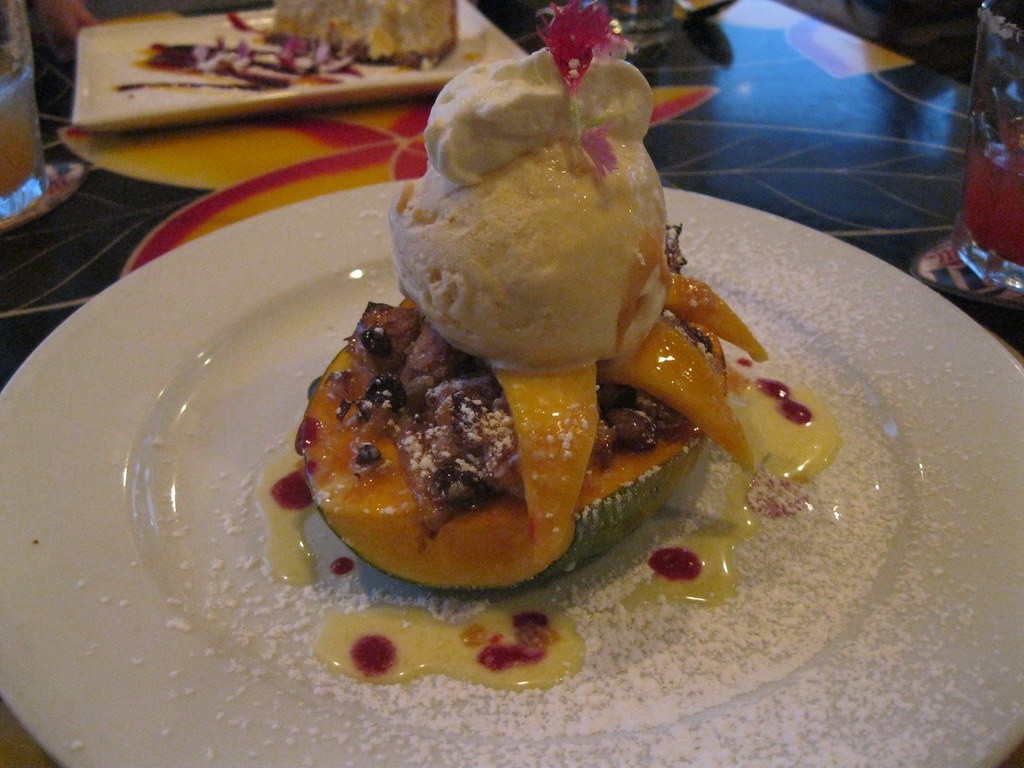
[382,0,673,368]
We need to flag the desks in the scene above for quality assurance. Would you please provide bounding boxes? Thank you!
[1,0,1024,765]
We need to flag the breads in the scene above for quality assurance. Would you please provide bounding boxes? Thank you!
[276,1,460,64]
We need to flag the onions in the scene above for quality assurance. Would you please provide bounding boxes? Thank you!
[190,10,361,83]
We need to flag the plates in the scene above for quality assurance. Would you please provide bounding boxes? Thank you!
[73,1,532,128]
[0,178,1024,768]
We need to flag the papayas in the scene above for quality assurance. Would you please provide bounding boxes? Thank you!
[305,294,729,596]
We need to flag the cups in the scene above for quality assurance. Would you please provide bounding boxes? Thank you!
[590,0,674,50]
[952,0,1024,293]
[0,1,49,221]
